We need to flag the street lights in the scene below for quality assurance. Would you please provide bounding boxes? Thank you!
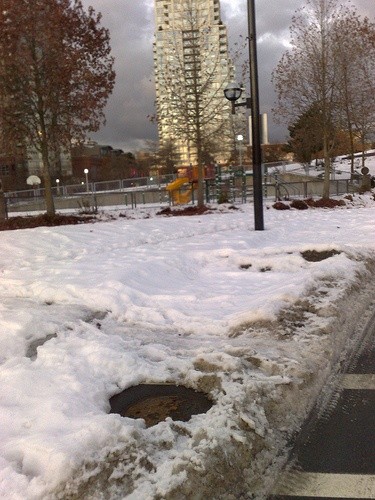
[81,168,90,194]
[225,0,266,231]
[149,176,155,186]
[55,178,61,197]
[235,134,243,167]
